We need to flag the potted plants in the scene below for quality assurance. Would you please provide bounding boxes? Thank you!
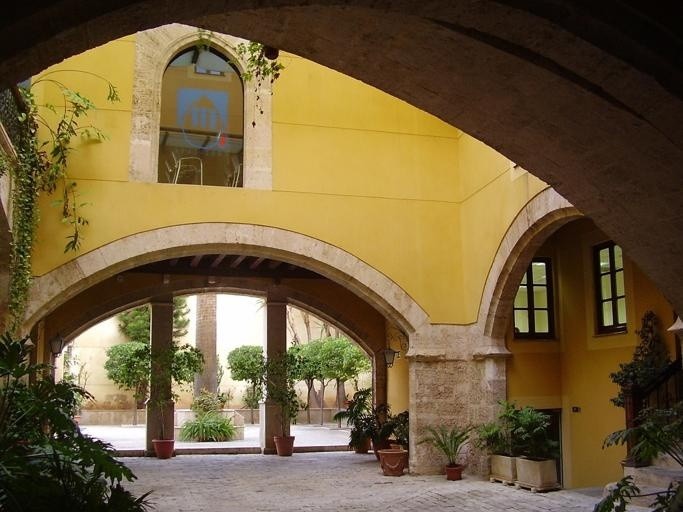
[179,409,237,443]
[332,385,410,477]
[267,380,310,457]
[415,421,475,481]
[145,376,182,460]
[476,400,560,493]
[227,41,289,128]
